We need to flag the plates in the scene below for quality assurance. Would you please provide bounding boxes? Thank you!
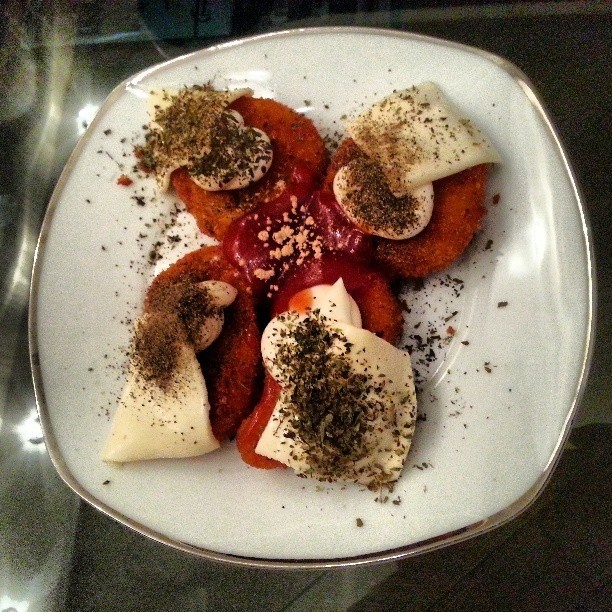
[27,25,599,572]
